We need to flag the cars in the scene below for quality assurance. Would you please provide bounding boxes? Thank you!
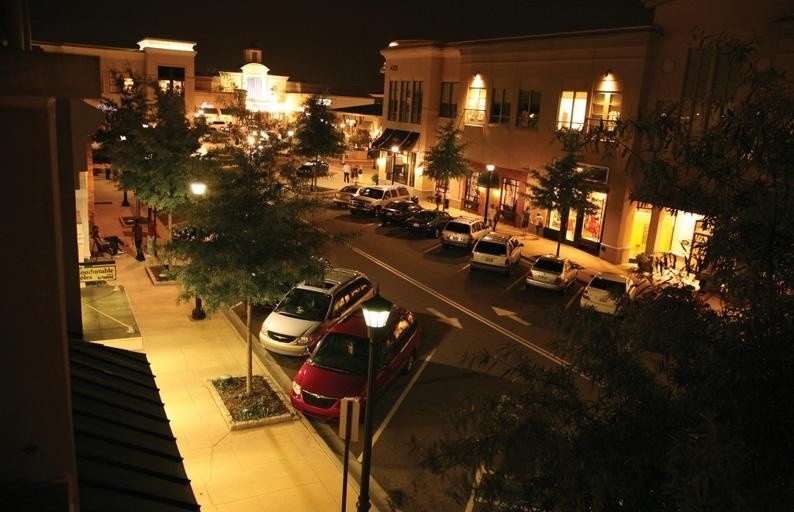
[296,159,329,178]
[580,270,638,317]
[406,210,454,238]
[333,182,364,208]
[469,231,523,275]
[289,300,420,425]
[440,219,492,251]
[380,200,424,225]
[526,254,578,294]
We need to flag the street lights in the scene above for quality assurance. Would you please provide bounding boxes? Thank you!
[356,283,394,512]
[258,265,375,358]
[191,176,207,320]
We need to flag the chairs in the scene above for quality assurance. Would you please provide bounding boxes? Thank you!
[91,236,113,263]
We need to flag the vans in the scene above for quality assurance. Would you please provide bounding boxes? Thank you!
[349,186,410,218]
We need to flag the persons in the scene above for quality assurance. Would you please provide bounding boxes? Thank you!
[492,206,500,232]
[435,190,441,211]
[487,203,496,229]
[519,206,532,236]
[534,213,543,239]
[130,218,145,261]
[343,162,363,183]
[91,225,127,253]
[91,232,124,257]
